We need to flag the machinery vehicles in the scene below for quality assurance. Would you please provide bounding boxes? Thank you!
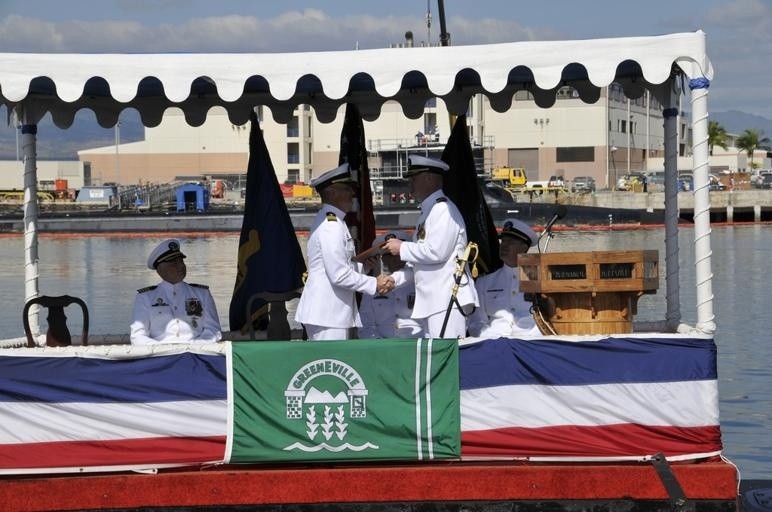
[490,166,528,194]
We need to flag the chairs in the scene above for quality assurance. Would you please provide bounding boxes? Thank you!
[245,291,311,341]
[23,294,89,347]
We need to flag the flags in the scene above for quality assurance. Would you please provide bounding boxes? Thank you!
[229,109,306,335]
[440,112,504,284]
[337,102,374,314]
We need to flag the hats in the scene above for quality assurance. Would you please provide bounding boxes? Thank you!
[147,239,186,269]
[403,155,450,178]
[372,231,409,247]
[496,218,538,246]
[311,162,357,193]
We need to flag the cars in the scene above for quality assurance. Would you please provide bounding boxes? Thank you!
[677,173,727,192]
[616,172,648,192]
[750,168,772,189]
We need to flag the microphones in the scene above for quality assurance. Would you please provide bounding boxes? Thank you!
[538,214,559,239]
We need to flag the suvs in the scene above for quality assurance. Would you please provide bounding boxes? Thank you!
[570,176,596,194]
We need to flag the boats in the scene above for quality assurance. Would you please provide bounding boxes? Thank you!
[0,28,743,512]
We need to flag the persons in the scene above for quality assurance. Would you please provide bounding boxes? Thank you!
[356,229,427,340]
[293,163,395,340]
[130,238,224,345]
[378,155,480,340]
[465,218,543,337]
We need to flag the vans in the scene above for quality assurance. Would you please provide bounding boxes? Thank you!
[547,175,569,193]
